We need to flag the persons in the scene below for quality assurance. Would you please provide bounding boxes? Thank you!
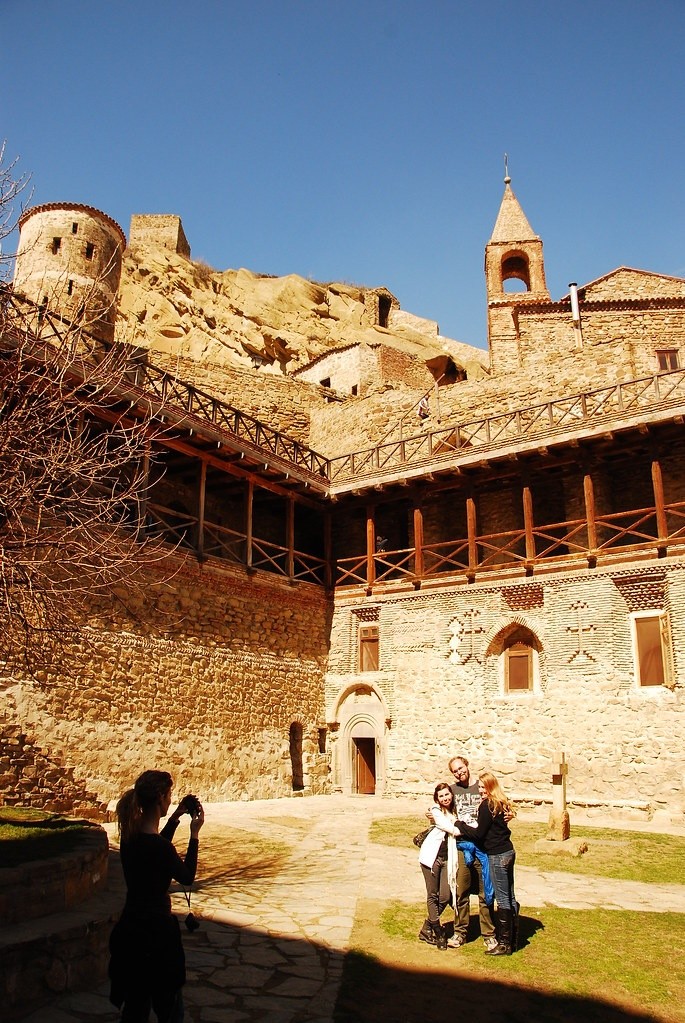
[418,756,519,956]
[107,771,204,1023]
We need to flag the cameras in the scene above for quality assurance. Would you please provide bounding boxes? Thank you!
[184,912,200,932]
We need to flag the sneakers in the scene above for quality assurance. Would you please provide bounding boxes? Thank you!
[483,938,498,951]
[447,934,466,947]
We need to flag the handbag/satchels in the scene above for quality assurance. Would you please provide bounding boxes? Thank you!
[412,826,435,848]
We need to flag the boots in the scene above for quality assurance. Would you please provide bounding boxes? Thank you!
[485,906,513,956]
[429,918,446,948]
[419,919,437,944]
[511,902,521,951]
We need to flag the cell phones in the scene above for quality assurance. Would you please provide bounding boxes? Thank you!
[183,796,199,819]
